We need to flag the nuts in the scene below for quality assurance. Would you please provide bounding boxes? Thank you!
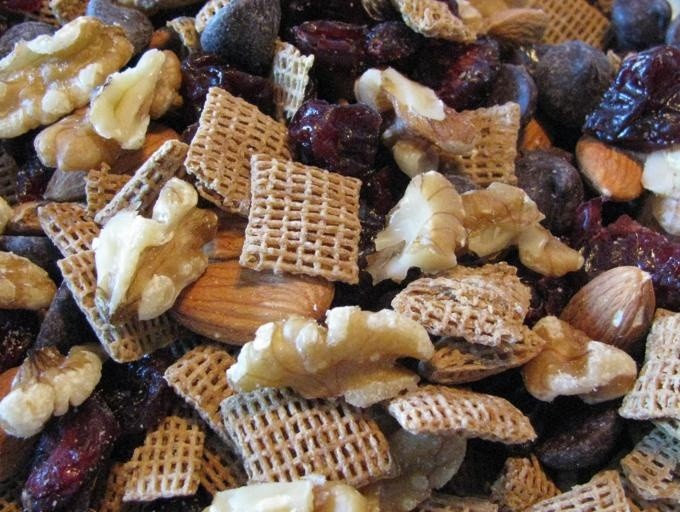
[0,0,680,512]
